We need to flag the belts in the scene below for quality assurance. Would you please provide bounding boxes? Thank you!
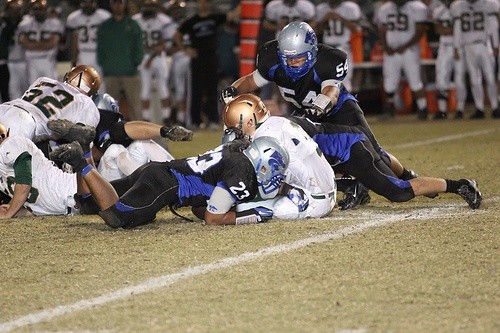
[311,191,334,199]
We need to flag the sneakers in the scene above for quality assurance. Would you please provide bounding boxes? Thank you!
[457,178,481,210]
[50,140,84,164]
[166,127,193,141]
[405,170,437,198]
[338,180,371,211]
[46,118,97,143]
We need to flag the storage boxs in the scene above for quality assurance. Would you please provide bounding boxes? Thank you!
[428,82,457,110]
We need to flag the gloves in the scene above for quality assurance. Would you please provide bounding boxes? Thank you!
[219,87,235,102]
[281,183,309,212]
[299,105,324,124]
[254,207,273,223]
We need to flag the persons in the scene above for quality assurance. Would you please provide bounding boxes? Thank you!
[0,64,193,219]
[219,21,482,210]
[191,94,337,219]
[47,119,309,229]
[0,0,500,129]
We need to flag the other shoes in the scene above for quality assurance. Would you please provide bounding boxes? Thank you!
[384,107,500,120]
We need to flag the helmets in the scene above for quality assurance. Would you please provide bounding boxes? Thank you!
[0,123,8,144]
[63,64,100,98]
[277,22,318,79]
[223,93,270,138]
[94,93,120,112]
[242,136,290,184]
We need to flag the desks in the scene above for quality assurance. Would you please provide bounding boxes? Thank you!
[351,59,435,122]
